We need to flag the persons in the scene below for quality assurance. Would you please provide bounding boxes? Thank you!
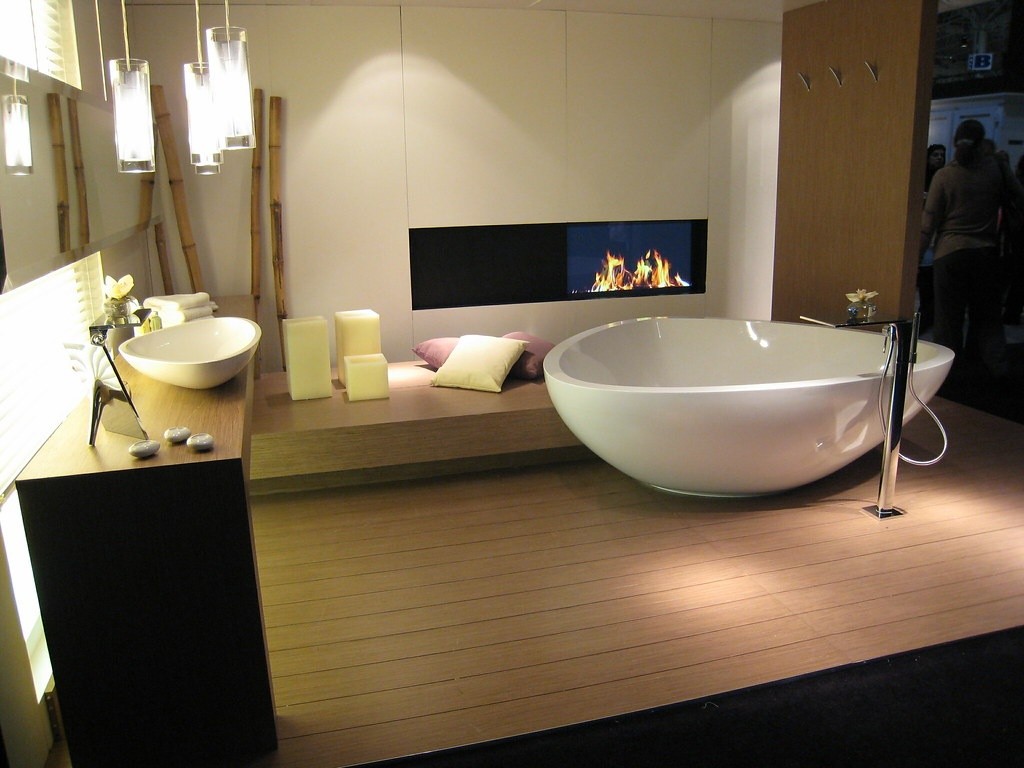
[918,118,1024,396]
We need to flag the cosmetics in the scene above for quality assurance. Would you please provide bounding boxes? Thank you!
[151,311,162,331]
[140,318,151,334]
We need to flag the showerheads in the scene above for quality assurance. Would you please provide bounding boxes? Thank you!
[908,311,921,363]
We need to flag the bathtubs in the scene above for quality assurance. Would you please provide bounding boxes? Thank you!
[543,315,956,499]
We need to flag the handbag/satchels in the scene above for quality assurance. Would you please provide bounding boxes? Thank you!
[998,159,1024,253]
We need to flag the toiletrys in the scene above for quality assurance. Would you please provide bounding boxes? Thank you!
[141,311,162,334]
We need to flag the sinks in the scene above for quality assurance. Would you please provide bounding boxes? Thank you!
[118,316,262,390]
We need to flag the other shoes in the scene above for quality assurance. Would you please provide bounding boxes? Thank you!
[1001,314,1020,325]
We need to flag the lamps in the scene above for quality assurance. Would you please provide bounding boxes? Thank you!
[108,0,256,176]
[0,57,33,176]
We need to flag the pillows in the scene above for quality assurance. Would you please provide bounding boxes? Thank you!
[431,334,530,392]
[409,336,460,372]
[501,331,556,382]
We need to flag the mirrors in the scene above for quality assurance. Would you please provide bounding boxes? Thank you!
[0,55,165,295]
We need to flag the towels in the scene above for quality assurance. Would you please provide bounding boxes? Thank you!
[143,292,214,328]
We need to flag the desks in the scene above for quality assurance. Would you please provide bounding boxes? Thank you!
[15,294,281,768]
